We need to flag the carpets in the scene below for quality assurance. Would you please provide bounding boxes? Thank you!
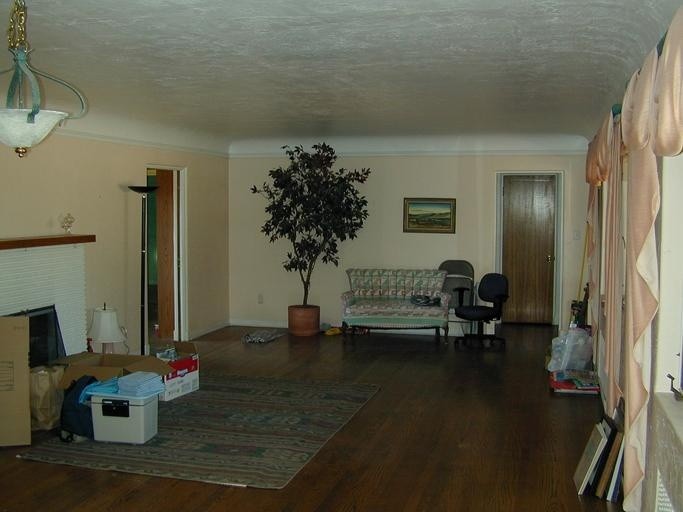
[15,374,382,489]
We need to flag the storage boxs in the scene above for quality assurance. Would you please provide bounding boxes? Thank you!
[0,315,32,446]
[54,352,176,393]
[90,393,159,445]
[156,340,200,401]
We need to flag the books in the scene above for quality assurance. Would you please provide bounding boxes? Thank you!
[573,396,625,503]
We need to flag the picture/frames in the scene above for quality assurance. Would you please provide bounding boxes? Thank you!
[572,396,626,502]
[403,196,456,234]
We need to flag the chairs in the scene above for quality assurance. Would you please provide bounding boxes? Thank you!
[452,272,509,351]
[437,260,475,336]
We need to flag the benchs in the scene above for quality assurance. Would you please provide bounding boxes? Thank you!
[340,268,451,346]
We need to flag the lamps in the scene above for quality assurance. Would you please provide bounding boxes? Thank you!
[128,185,160,357]
[86,303,126,354]
[0,0,88,156]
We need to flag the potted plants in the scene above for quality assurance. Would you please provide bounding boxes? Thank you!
[248,141,371,336]
